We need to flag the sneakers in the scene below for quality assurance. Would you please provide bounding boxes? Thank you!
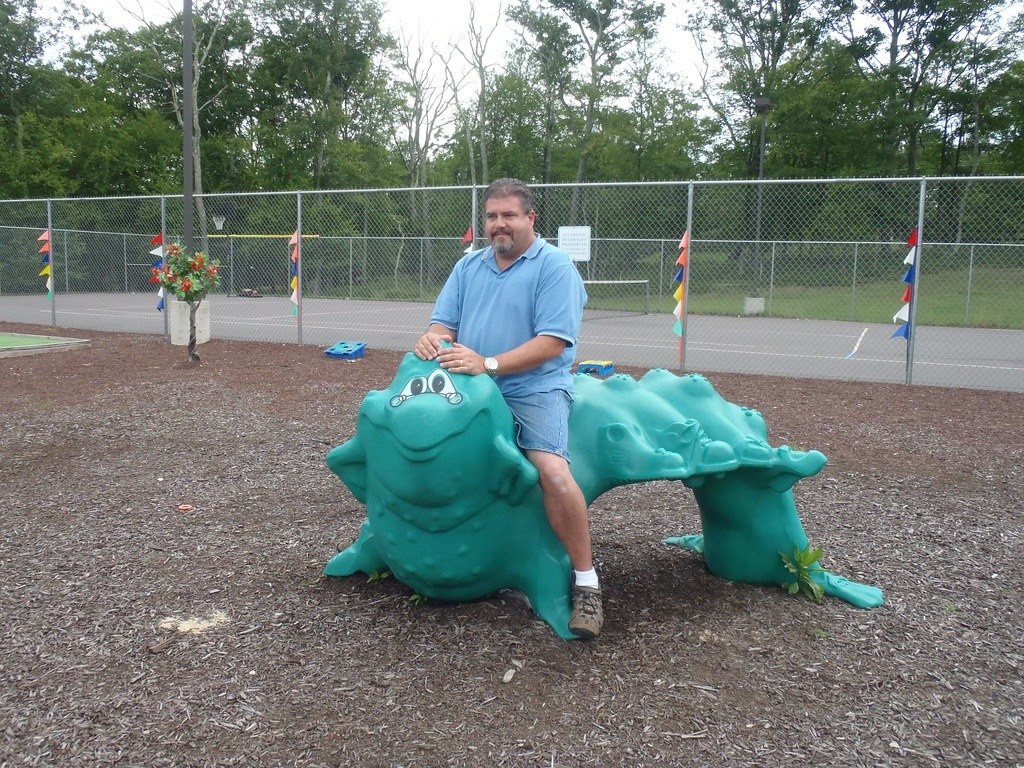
[337,539,356,553]
[569,583,606,638]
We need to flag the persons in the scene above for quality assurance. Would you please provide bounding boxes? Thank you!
[411,178,605,638]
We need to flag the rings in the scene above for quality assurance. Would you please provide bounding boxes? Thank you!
[461,360,462,367]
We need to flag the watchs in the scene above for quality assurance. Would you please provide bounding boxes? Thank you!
[484,358,499,377]
